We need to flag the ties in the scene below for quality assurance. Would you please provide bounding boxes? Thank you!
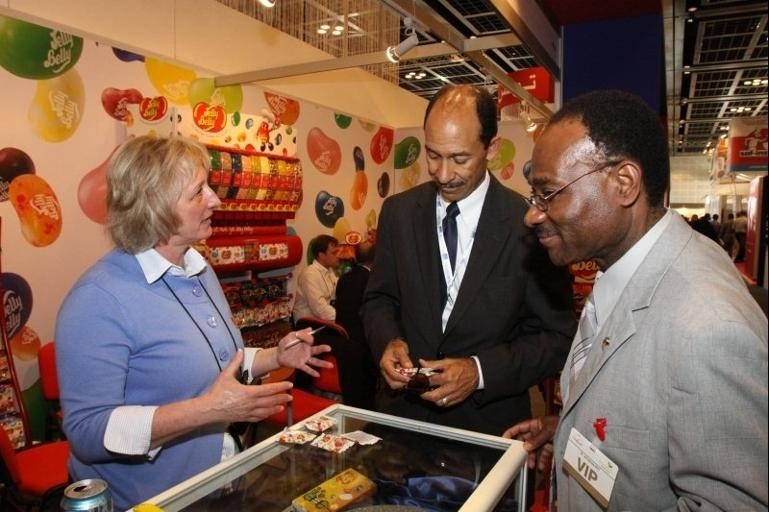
[566,289,599,401]
[440,199,463,277]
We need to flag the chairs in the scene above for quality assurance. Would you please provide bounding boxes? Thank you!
[0,342,73,512]
[245,316,354,445]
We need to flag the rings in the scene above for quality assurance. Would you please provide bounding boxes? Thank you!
[440,394,450,405]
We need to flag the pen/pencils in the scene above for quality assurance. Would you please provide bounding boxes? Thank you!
[282,325,328,350]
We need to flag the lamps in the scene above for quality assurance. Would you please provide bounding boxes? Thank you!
[387,25,419,64]
[520,102,535,132]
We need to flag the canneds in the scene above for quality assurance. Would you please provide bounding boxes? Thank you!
[59,478,116,512]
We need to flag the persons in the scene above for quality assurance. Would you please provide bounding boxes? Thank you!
[356,82,576,512]
[54,134,336,512]
[357,420,506,511]
[332,240,380,362]
[287,230,340,334]
[502,88,767,512]
[676,209,750,265]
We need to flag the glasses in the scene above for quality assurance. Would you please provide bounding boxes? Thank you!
[519,157,620,214]
